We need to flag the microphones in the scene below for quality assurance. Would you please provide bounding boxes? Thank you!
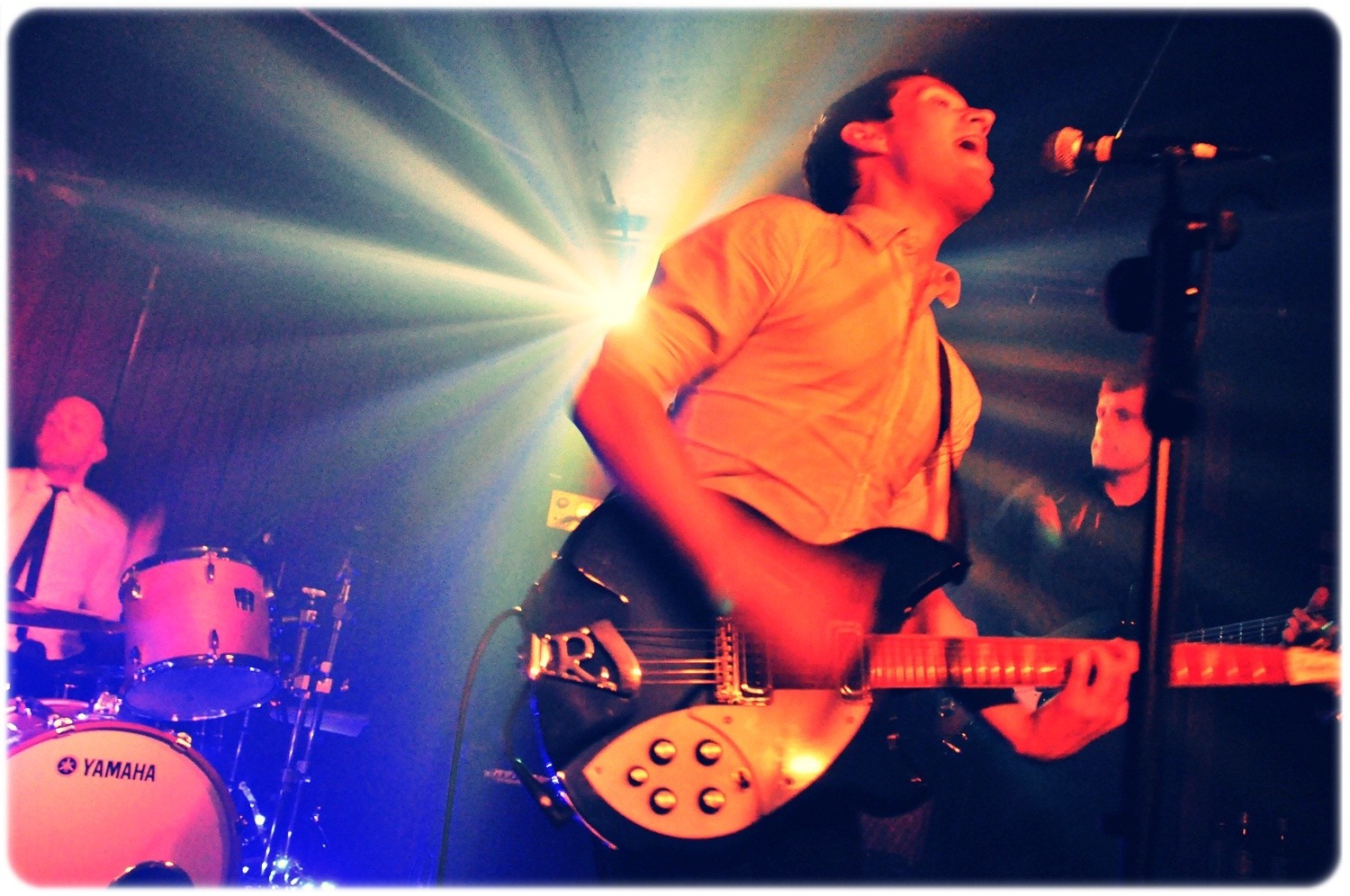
[1043,126,1256,176]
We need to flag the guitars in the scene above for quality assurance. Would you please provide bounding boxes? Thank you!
[823,606,1339,816]
[525,488,1342,854]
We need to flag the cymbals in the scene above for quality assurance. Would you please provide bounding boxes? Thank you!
[7,600,136,635]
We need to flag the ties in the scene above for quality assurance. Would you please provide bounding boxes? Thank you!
[6,484,70,641]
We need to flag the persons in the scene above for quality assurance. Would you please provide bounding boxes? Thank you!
[956,357,1339,888]
[516,70,1131,888]
[5,395,127,691]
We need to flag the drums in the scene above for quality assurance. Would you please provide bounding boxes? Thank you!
[119,546,279,721]
[7,692,240,889]
